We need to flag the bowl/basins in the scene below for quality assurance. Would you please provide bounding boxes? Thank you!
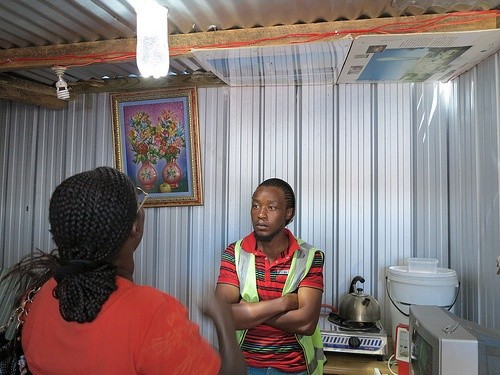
[407,258,439,274]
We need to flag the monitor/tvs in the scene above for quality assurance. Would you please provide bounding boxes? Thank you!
[408,304,500,375]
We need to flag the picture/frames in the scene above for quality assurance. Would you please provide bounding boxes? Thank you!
[110,87,204,207]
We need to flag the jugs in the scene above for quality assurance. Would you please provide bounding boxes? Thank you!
[338,276,381,322]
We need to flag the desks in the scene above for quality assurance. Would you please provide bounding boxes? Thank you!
[323,353,398,375]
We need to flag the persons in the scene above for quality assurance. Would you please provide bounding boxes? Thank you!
[215,178,324,375]
[21,167,246,375]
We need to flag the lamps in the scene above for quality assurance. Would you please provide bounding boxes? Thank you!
[52,65,71,102]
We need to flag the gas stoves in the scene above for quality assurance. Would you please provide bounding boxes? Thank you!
[318,311,388,361]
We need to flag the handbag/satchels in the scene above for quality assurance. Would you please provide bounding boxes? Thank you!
[0,273,51,375]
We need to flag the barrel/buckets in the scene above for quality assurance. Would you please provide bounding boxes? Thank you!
[385,265,460,349]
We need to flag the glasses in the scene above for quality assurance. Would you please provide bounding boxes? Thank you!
[136,186,149,216]
